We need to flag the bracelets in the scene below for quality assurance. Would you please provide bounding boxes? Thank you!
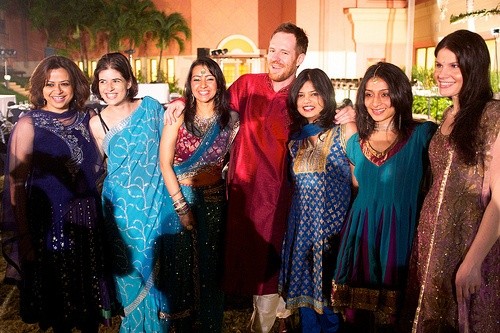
[170,188,191,217]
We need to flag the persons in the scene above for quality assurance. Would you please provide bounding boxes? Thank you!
[288,68,361,333]
[162,22,357,333]
[406,29,500,333]
[159,57,241,333]
[6,55,112,332]
[87,52,169,333]
[330,61,439,333]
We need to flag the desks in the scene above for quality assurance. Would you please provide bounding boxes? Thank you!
[7,103,33,124]
[0,95,16,118]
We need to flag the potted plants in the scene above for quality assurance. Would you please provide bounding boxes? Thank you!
[411,64,500,124]
